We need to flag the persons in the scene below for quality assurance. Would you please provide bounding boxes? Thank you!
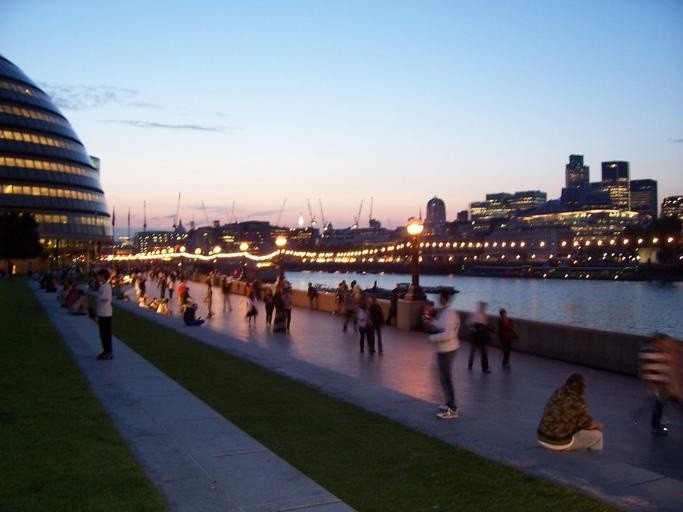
[538,373,606,452]
[307,283,319,309]
[28,265,100,316]
[641,335,670,435]
[244,272,293,334]
[420,287,520,420]
[333,280,400,354]
[94,269,114,360]
[115,267,233,326]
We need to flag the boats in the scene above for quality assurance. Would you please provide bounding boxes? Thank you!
[360,282,460,300]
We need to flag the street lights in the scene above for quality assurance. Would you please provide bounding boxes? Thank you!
[275,235,288,280]
[404,219,427,302]
[106,242,250,281]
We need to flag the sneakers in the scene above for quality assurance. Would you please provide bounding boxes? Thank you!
[650,423,669,434]
[96,350,114,360]
[436,404,460,419]
